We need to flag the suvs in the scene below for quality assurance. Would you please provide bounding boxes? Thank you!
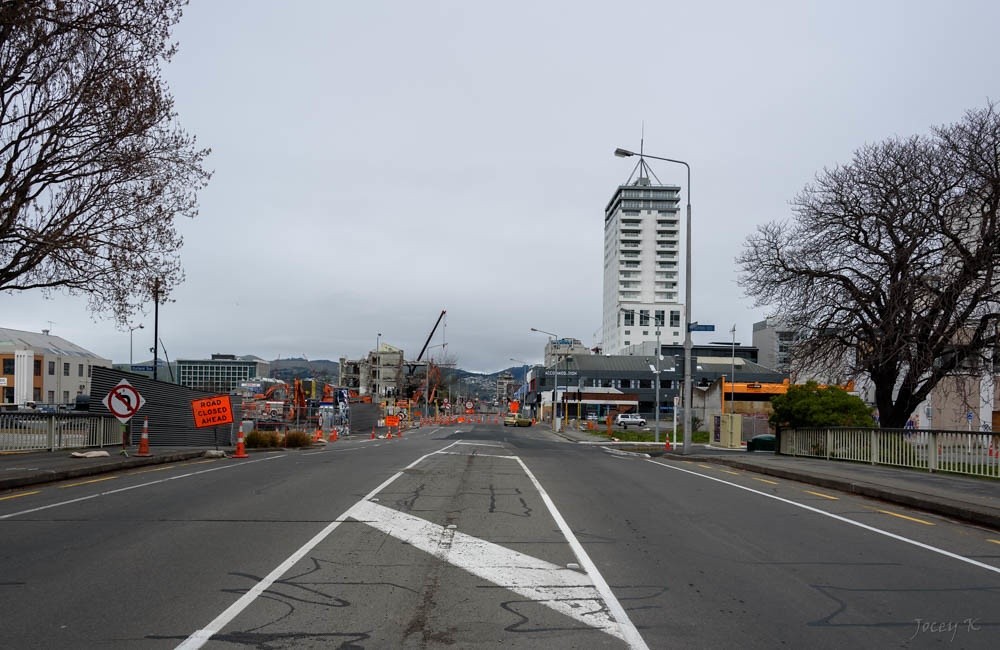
[615,413,647,427]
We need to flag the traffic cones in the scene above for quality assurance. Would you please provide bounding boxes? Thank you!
[994,449,1000,458]
[662,432,673,451]
[132,416,155,457]
[387,427,393,439]
[231,421,250,458]
[467,414,500,425]
[988,440,994,456]
[371,425,377,439]
[397,426,405,438]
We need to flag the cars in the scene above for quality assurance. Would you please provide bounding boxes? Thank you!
[585,412,599,421]
[503,412,532,427]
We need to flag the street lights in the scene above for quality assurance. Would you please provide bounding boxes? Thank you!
[565,356,574,427]
[375,333,382,403]
[130,324,144,370]
[614,149,694,455]
[621,309,661,443]
[531,328,558,420]
[510,358,526,410]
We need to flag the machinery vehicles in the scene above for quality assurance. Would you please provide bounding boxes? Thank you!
[254,378,350,429]
